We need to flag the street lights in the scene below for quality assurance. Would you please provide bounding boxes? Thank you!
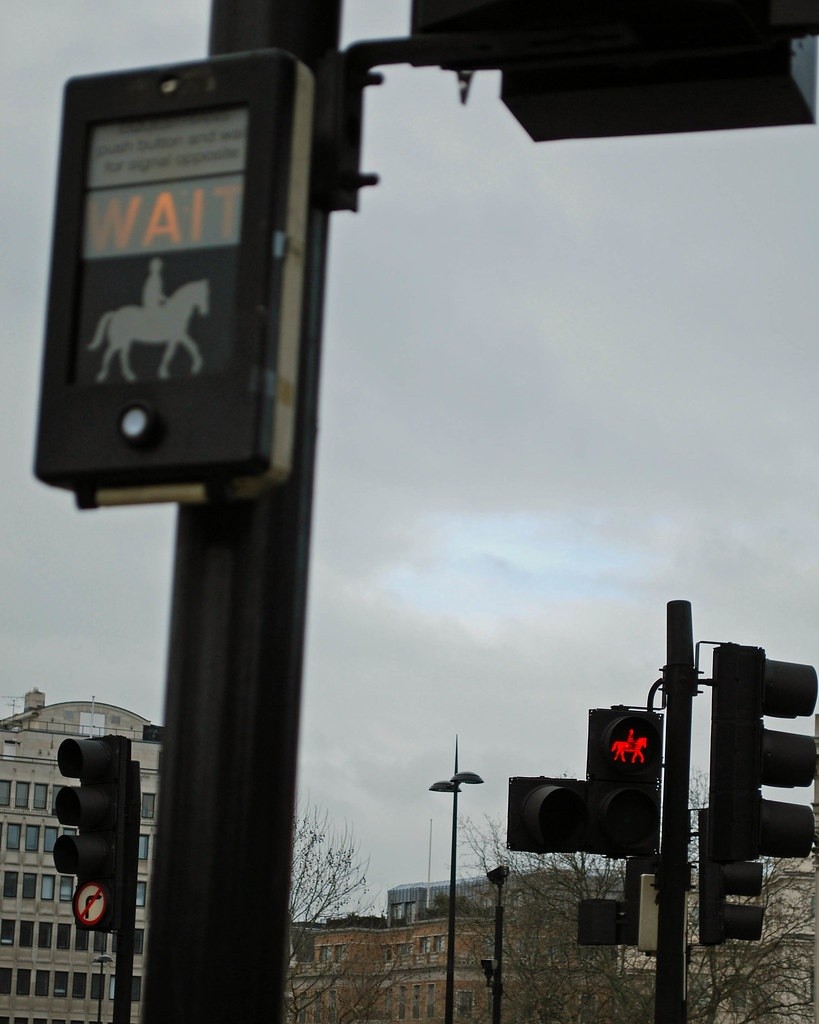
[428,732,485,1024]
[487,866,510,1024]
[92,933,114,1024]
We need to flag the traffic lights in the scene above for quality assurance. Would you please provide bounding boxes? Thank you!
[708,640,818,863]
[698,806,765,945]
[587,702,664,784]
[53,734,120,879]
[506,776,662,858]
